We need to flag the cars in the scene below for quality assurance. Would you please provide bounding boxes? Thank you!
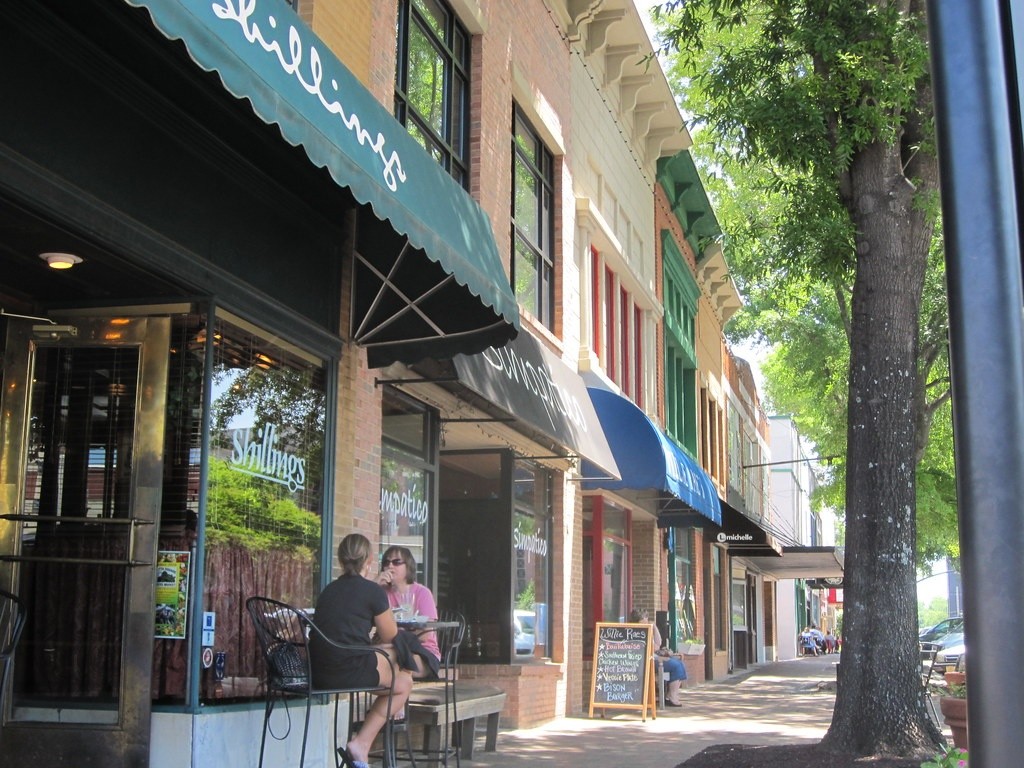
[919,616,965,674]
[513,608,536,655]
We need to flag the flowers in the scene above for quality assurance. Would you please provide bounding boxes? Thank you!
[920,748,969,768]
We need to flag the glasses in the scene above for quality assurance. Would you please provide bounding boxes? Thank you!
[383,559,406,567]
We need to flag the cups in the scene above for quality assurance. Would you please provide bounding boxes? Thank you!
[399,592,415,620]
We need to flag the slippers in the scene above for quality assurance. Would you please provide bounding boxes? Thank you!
[337,747,369,768]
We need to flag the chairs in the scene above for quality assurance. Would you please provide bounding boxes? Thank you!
[246,595,466,768]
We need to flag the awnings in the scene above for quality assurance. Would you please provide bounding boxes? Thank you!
[122,1,523,370]
[581,387,843,581]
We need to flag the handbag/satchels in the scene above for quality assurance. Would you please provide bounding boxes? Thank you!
[270,641,308,692]
[656,650,671,657]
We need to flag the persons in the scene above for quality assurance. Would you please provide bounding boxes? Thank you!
[372,545,441,724]
[631,607,687,706]
[799,623,841,657]
[309,533,413,768]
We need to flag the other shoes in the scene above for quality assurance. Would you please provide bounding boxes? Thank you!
[665,699,682,707]
[366,710,405,724]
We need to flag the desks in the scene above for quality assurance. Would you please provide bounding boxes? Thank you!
[264,621,459,768]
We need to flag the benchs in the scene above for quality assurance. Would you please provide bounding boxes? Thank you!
[655,654,683,711]
[383,683,507,768]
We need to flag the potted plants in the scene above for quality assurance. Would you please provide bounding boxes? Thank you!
[940,683,968,750]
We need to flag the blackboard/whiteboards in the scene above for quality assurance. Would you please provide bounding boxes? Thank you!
[590,622,653,710]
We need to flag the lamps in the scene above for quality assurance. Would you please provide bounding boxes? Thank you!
[39,253,83,269]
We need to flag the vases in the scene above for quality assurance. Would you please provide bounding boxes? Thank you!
[944,672,966,694]
